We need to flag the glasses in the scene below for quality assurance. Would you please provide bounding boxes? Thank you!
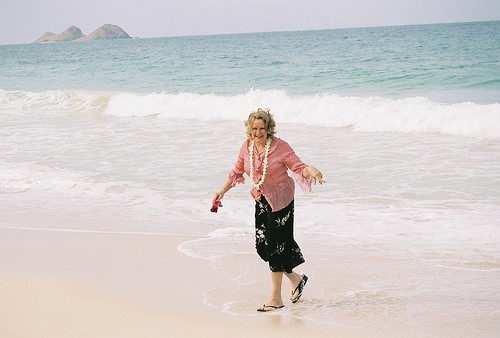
[211,198,222,213]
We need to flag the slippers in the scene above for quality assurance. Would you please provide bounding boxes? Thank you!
[291,274,308,303]
[257,304,284,313]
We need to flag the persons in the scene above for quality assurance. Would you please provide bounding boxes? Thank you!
[214,108,326,312]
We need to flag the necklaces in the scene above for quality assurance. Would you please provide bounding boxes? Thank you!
[249,136,273,188]
